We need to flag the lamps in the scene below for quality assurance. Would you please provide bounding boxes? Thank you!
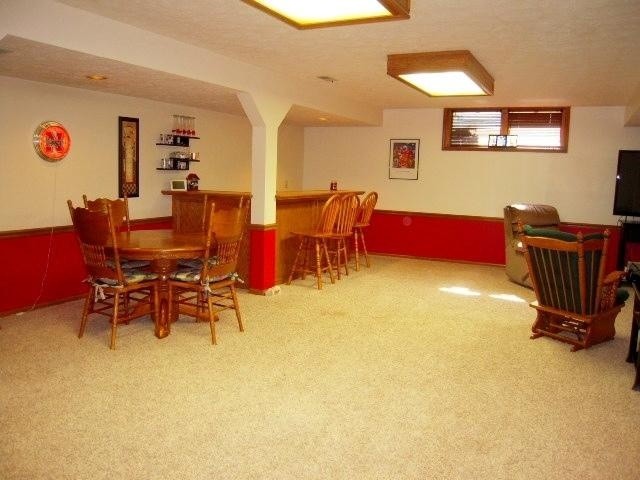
[517,221,629,352]
[285,191,378,290]
[67,195,250,350]
[504,204,561,289]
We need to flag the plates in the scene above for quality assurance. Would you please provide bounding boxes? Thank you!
[156,135,200,170]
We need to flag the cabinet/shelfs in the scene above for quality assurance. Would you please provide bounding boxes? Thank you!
[170,114,197,138]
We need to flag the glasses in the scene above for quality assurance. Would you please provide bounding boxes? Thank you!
[242,0,410,30]
[387,50,494,97]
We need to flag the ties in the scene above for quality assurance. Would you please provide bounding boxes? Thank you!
[612,149,640,216]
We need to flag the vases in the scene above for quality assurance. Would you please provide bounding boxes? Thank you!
[186,173,200,181]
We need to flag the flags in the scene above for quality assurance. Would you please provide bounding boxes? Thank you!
[189,181,198,191]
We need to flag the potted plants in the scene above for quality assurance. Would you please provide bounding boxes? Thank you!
[389,139,420,180]
[119,116,139,198]
